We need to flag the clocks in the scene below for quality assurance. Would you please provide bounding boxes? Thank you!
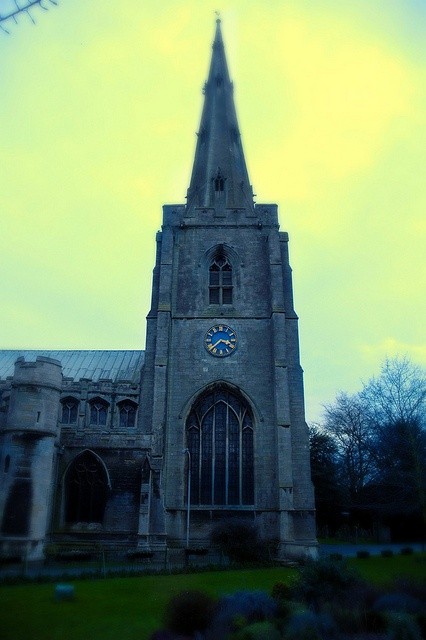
[203,324,238,357]
